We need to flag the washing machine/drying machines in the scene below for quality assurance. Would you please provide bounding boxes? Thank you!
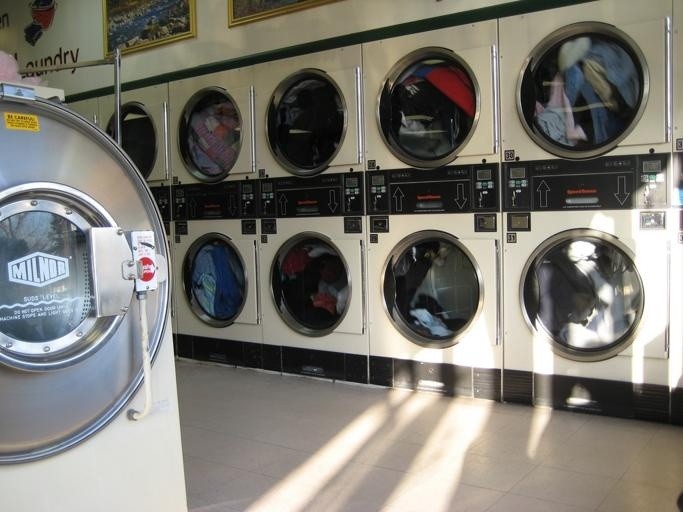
[0,1,682,512]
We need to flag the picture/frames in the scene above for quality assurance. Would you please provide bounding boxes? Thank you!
[102,0,197,59]
[227,0,345,28]
